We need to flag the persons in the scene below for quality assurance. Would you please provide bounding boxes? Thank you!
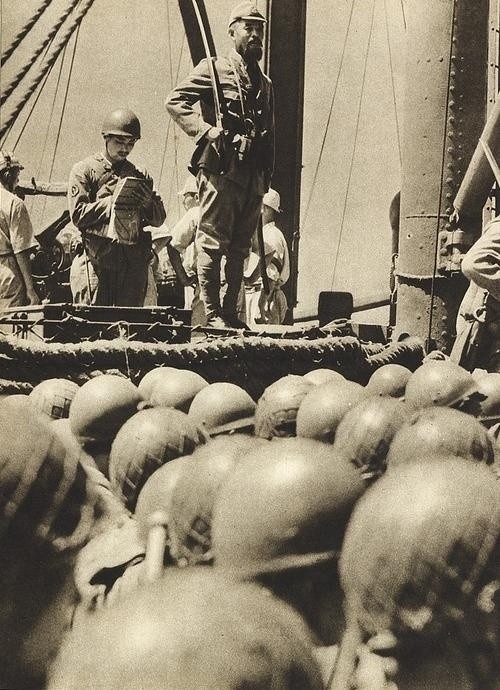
[450,214,500,375]
[165,1,276,333]
[2,334,500,690]
[66,107,166,308]
[0,148,42,317]
[58,174,291,329]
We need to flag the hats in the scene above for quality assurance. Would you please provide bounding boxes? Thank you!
[262,188,282,213]
[229,2,267,27]
[177,176,199,196]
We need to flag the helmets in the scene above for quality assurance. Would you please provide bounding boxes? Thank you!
[102,106,141,138]
[1,150,24,172]
